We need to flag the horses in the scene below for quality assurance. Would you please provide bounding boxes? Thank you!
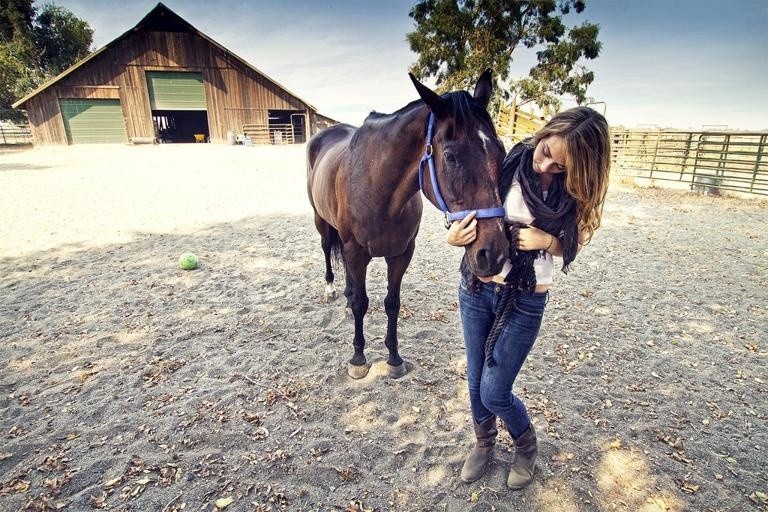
[305,66,513,381]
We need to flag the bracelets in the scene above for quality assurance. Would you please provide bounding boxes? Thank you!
[544,235,554,252]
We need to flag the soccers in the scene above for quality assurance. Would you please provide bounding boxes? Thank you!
[178,252,199,270]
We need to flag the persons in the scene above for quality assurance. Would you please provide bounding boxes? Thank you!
[443,103,612,493]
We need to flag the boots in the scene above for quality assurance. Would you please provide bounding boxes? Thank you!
[462,414,498,482]
[507,421,539,489]
